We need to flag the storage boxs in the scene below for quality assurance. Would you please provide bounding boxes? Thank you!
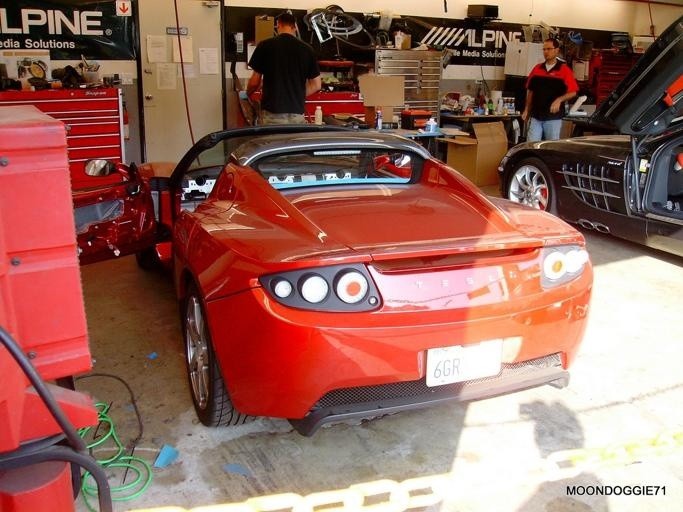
[439,122,508,186]
[357,76,406,123]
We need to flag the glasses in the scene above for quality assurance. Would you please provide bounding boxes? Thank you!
[542,47,557,51]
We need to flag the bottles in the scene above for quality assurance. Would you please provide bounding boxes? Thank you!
[487,99,493,115]
[314,106,323,126]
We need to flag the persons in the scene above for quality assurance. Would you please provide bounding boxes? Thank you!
[245,11,322,127]
[521,36,579,145]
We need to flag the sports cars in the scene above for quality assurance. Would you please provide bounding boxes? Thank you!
[138,123,592,438]
[499,15,683,258]
